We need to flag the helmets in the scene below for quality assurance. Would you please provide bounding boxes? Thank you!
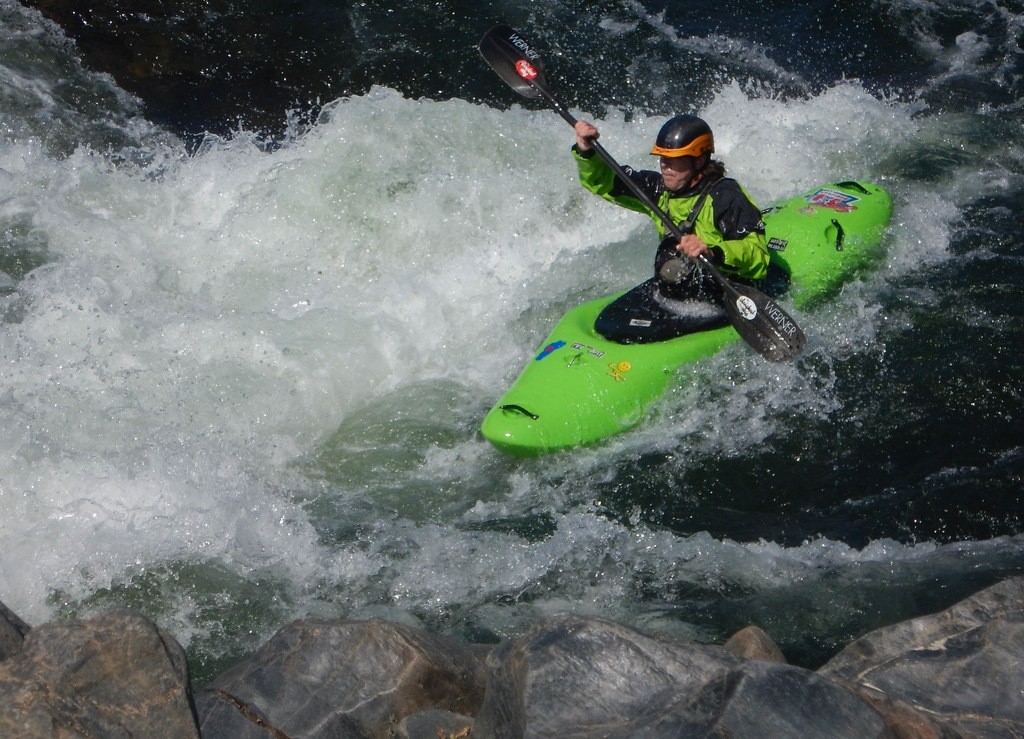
[650,115,716,157]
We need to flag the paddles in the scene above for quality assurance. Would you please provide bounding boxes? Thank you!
[478,24,806,364]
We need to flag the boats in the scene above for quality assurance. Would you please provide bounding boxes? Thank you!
[480,180,894,456]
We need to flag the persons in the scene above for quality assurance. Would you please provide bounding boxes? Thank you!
[571,113,771,288]
